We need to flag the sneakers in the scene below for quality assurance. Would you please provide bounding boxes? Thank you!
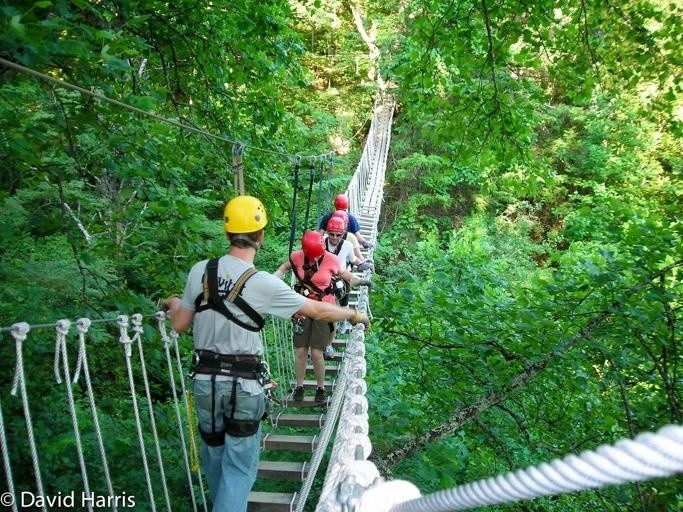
[316,386,326,401]
[293,386,305,400]
[326,345,334,359]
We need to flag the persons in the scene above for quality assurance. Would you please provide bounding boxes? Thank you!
[318,192,376,250]
[272,231,371,402]
[320,216,375,360]
[157,193,374,512]
[329,207,375,334]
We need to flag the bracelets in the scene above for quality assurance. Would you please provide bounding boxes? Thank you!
[350,309,359,327]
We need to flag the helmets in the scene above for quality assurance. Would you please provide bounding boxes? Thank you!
[335,195,348,209]
[304,232,326,259]
[224,196,268,234]
[327,217,344,233]
[333,210,349,222]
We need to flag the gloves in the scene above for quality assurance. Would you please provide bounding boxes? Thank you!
[360,279,370,286]
[363,241,373,248]
[353,311,369,327]
[358,263,372,271]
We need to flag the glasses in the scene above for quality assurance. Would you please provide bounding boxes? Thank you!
[330,233,341,236]
